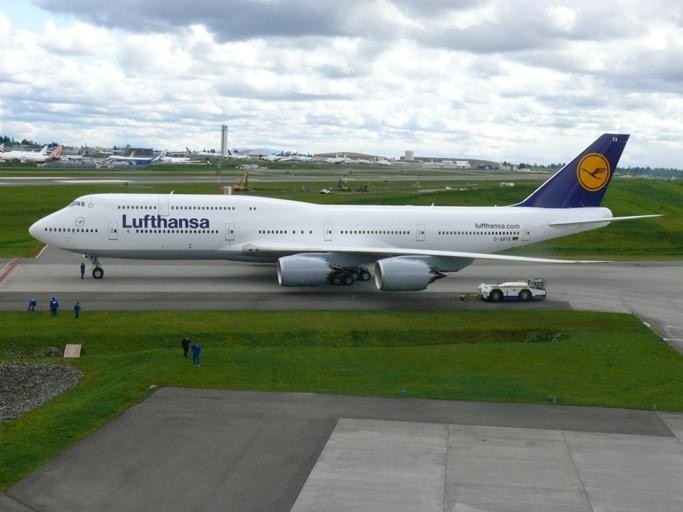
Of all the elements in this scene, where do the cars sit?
[320,188,331,194]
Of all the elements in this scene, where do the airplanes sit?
[27,133,666,292]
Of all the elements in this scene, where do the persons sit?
[73,301,81,317]
[190,340,201,367]
[27,297,38,311]
[338,173,371,192]
[50,296,59,317]
[181,334,190,357]
[80,260,85,279]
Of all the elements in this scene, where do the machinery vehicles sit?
[476,277,547,303]
